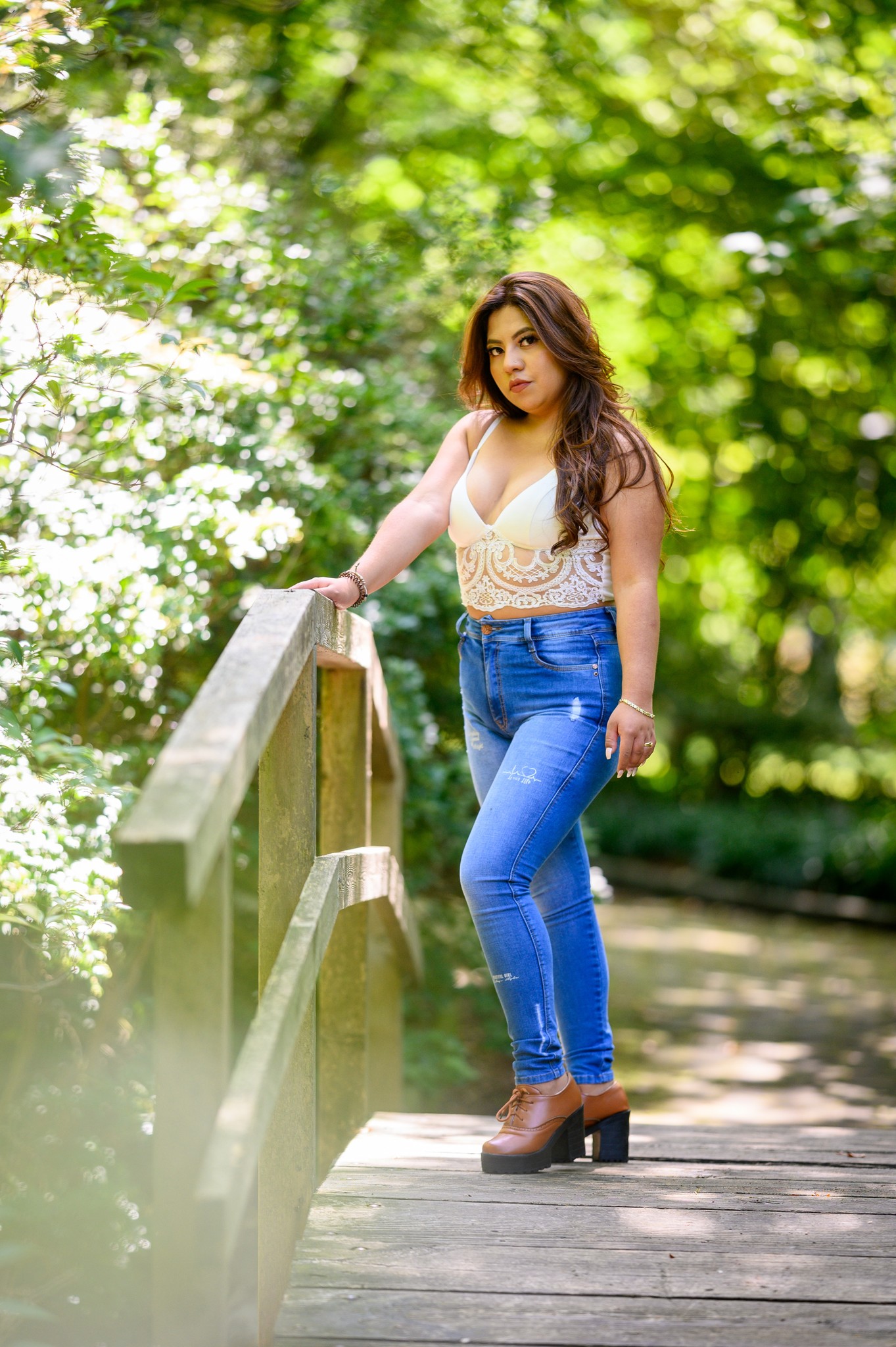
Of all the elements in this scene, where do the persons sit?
[284,272,694,1175]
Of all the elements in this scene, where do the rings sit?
[644,741,653,748]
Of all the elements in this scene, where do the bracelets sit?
[618,699,655,719]
[338,562,368,608]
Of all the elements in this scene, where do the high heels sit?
[481,1071,586,1174]
[582,1082,631,1163]
